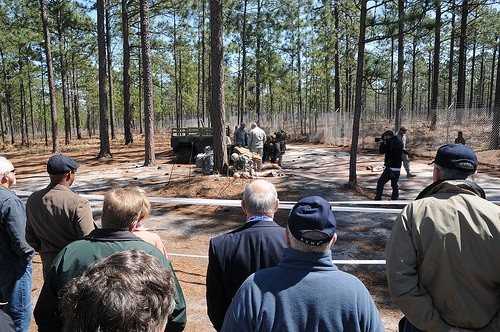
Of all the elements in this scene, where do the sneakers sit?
[407,174,416,178]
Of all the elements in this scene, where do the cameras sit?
[375,138,383,142]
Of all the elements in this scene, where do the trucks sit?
[170,124,231,165]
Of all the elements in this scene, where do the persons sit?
[202,122,286,176]
[0,153,187,332]
[221,195,384,332]
[394,127,416,178]
[371,130,404,206]
[206,179,288,332]
[386,143,500,332]
[455,131,465,145]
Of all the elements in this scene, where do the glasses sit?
[400,129,407,132]
[10,170,14,173]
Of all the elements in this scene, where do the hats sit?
[47,153,80,175]
[251,123,257,128]
[427,143,477,172]
[287,196,336,246]
[400,126,409,131]
[0,157,14,177]
[241,123,246,126]
